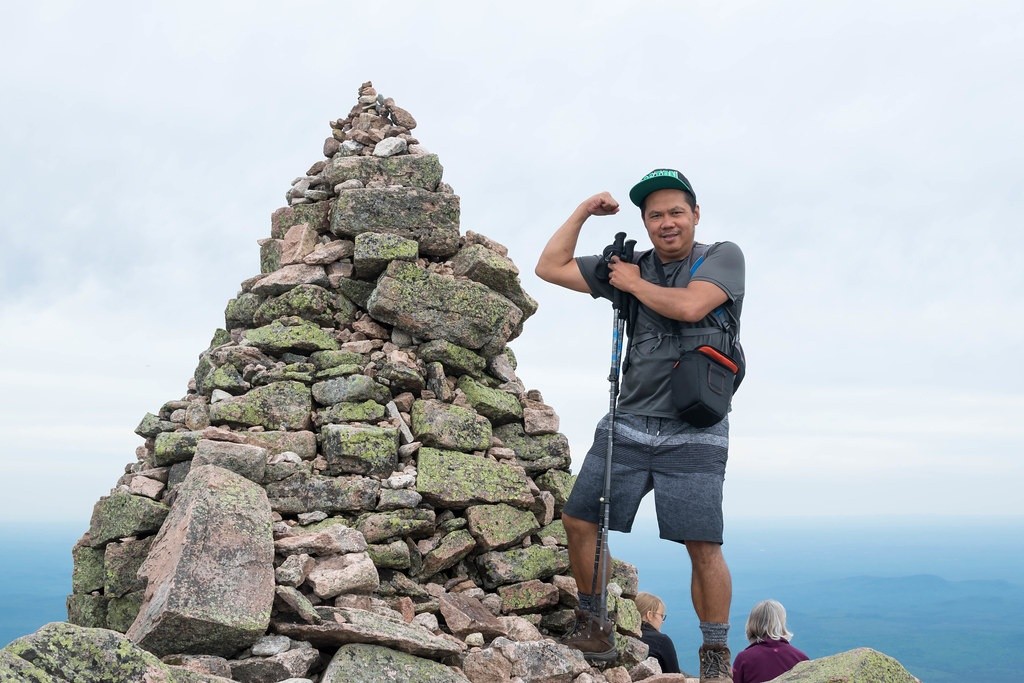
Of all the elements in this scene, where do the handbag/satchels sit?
[671,344,740,429]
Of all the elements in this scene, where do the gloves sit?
[595,232,637,281]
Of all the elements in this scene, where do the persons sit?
[633,592,681,673]
[535,168,746,683]
[732,600,809,683]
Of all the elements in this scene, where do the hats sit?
[629,168,696,209]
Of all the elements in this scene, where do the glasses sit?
[651,611,667,622]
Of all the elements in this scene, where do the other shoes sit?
[559,606,618,661]
[699,643,734,683]
